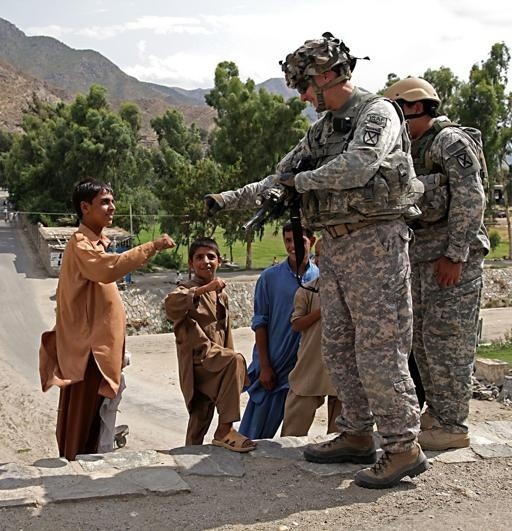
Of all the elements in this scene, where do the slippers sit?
[212,429,256,452]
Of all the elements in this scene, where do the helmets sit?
[382,77,442,110]
[282,33,358,88]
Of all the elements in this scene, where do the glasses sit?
[296,80,310,93]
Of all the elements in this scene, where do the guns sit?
[241,156,314,231]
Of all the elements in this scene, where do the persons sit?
[382,76,492,490]
[40,177,176,461]
[165,237,256,452]
[203,33,430,489]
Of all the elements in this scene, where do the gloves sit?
[205,194,224,216]
[279,172,295,188]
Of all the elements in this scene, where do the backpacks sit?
[457,125,490,205]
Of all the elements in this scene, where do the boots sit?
[304,432,376,465]
[415,411,469,450]
[354,446,430,489]
